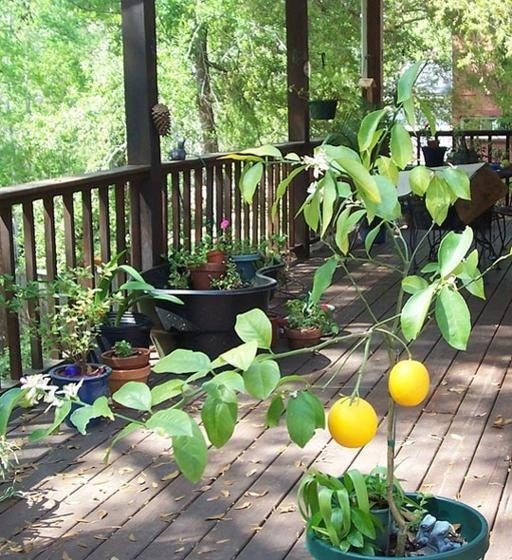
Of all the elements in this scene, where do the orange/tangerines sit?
[388,360,430,407]
[327,397,379,448]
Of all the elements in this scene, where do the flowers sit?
[0,51,512,559]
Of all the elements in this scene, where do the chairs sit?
[340,152,512,279]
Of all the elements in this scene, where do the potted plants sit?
[448,120,481,166]
[289,80,347,122]
[290,459,421,557]
[71,218,339,410]
[420,122,448,167]
[314,91,409,247]
[2,264,112,429]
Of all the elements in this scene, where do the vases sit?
[295,487,491,560]
